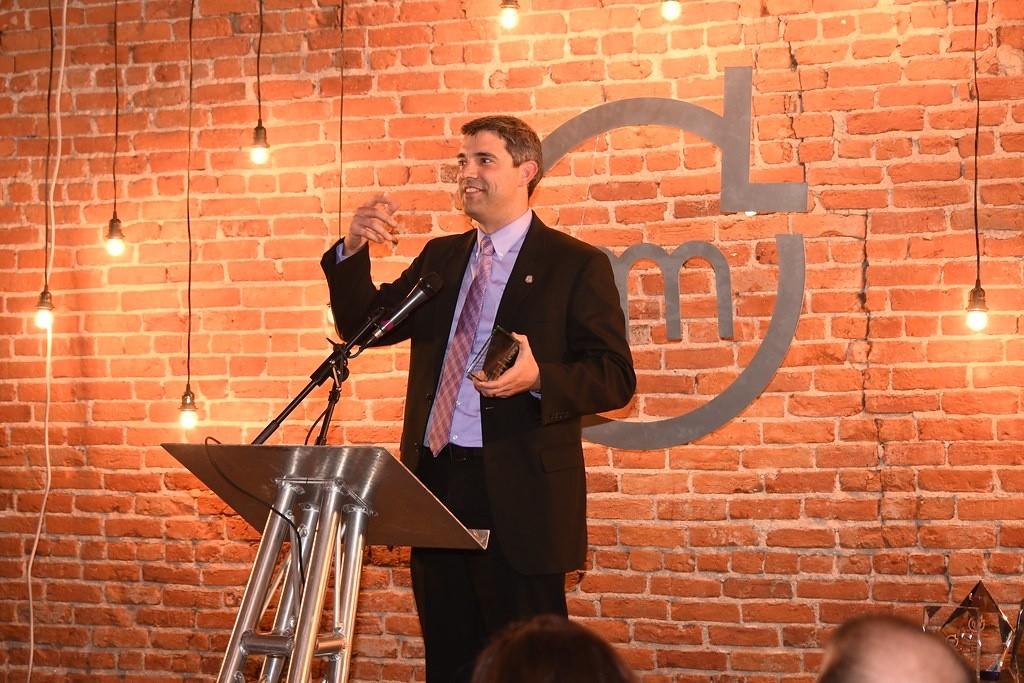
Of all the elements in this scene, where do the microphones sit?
[359,272,443,353]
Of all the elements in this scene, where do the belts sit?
[422,444,483,464]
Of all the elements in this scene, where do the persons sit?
[467,614,639,683]
[322,115,639,683]
[812,614,972,683]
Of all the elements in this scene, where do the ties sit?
[427,237,499,457]
